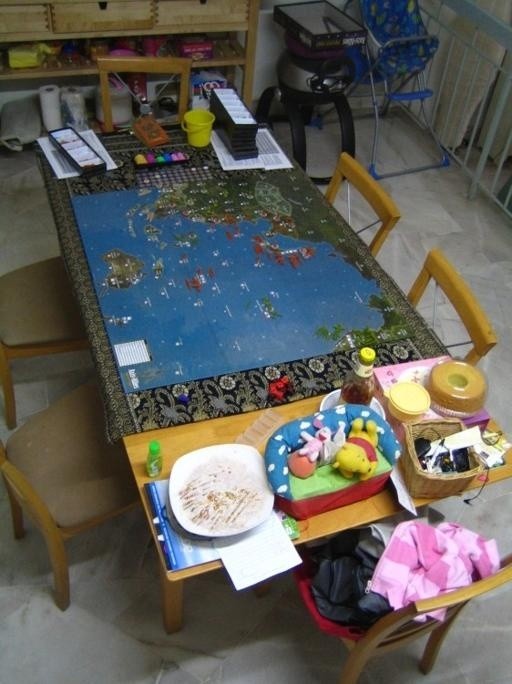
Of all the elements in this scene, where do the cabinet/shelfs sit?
[0,0,260,109]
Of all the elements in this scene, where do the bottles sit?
[145,440,163,480]
[336,346,378,407]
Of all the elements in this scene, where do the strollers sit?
[309,0,451,181]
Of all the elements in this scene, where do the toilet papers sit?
[61,86,83,125]
[39,85,62,131]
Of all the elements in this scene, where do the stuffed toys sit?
[264,404,404,521]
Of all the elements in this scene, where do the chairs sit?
[0,383,142,612]
[96,56,192,132]
[294,531,512,684]
[407,249,498,367]
[0,255,90,430]
[325,153,401,259]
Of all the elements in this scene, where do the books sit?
[143,478,301,574]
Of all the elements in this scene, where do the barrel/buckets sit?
[180,108,215,147]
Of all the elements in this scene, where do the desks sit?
[34,123,512,635]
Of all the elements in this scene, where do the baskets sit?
[401,418,484,498]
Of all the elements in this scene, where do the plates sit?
[165,442,277,540]
[319,388,386,422]
